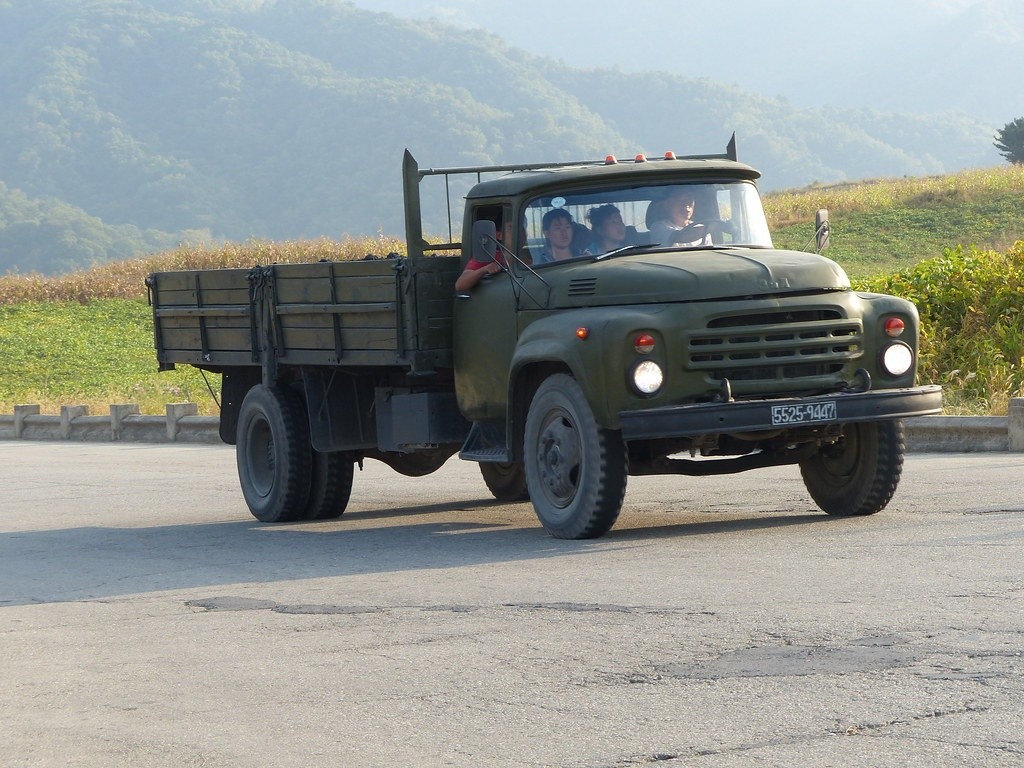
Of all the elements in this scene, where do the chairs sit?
[618,226,640,247]
[644,200,667,230]
[546,222,595,250]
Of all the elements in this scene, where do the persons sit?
[456,210,535,291]
[536,204,626,264]
[649,186,737,248]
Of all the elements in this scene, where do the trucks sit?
[148,131,945,539]
